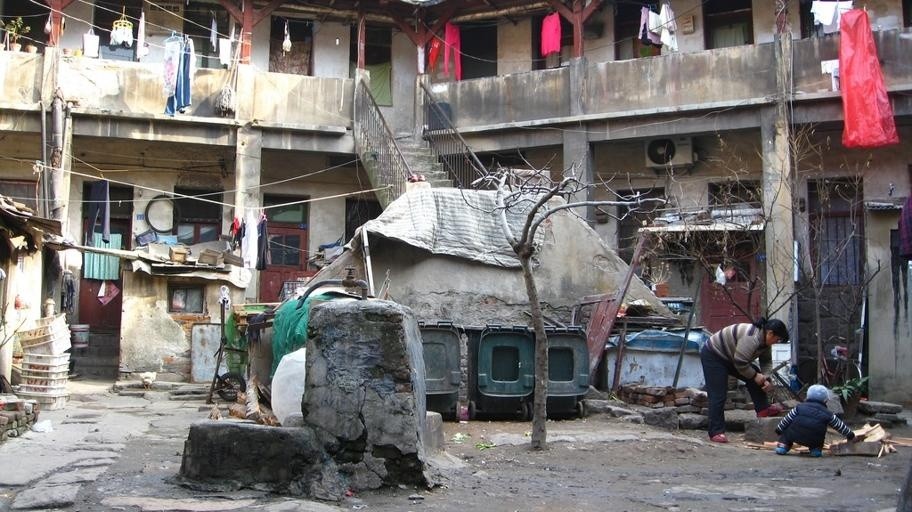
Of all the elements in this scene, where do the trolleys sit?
[204,292,251,407]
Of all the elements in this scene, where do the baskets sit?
[17,351,72,393]
[15,311,73,355]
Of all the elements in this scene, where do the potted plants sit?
[4,16,31,51]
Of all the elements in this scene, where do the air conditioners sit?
[643,134,694,170]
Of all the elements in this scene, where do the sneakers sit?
[775,446,787,455]
[710,434,729,443]
[809,448,822,456]
[755,403,782,417]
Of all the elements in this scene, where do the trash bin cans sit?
[417,320,591,423]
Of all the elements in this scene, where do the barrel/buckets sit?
[69,324,91,349]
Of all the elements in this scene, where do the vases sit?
[64,48,72,56]
[25,45,38,54]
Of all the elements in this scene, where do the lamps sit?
[335,36,340,46]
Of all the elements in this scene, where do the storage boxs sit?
[199,248,224,266]
[169,246,187,262]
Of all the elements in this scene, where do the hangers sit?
[208,9,217,19]
[162,30,190,47]
[87,24,94,35]
[641,0,670,14]
[546,7,560,16]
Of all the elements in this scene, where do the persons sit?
[700,317,790,443]
[774,385,857,457]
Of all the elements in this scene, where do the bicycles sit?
[797,331,865,390]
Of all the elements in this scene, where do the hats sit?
[807,384,830,404]
